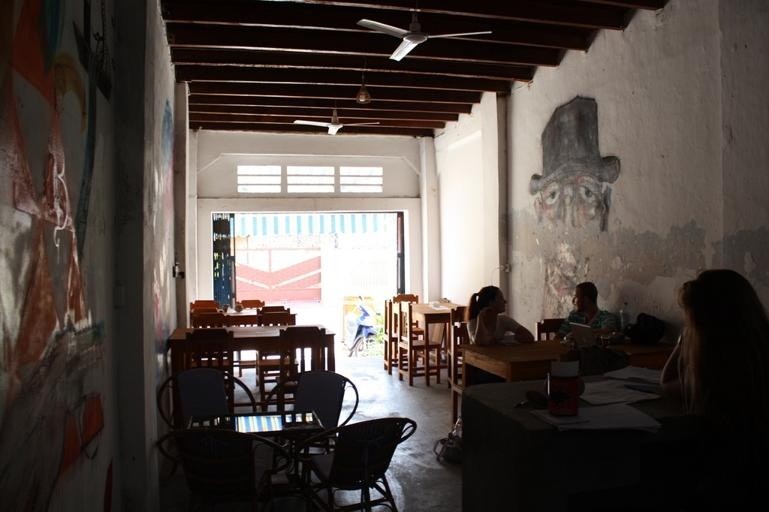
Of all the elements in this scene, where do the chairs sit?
[168,301,335,371]
[264,370,360,426]
[294,416,415,512]
[155,426,292,508]
[379,290,468,387]
[153,367,259,424]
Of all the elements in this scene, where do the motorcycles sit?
[340,296,380,359]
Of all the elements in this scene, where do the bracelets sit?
[678,334,682,345]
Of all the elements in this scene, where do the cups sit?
[504,331,516,344]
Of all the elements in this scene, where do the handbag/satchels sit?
[433,432,463,458]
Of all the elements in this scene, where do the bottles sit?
[620,301,632,328]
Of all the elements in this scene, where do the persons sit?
[659,269,769,512]
[554,282,621,376]
[467,287,534,383]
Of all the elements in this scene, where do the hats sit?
[528,96,620,195]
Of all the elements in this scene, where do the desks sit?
[453,338,667,423]
[460,365,691,511]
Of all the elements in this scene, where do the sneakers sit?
[452,418,463,439]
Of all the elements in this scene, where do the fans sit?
[293,109,382,138]
[356,11,495,65]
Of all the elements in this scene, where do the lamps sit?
[355,71,374,106]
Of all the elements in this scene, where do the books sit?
[568,322,592,337]
